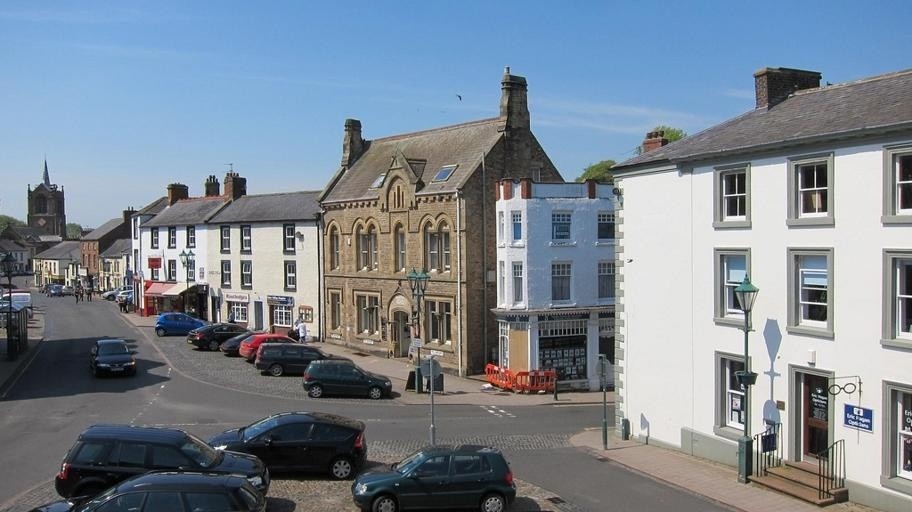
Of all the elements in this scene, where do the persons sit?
[23,277,27,288]
[79,286,84,302]
[294,315,302,327]
[118,296,123,313]
[86,285,92,302]
[229,310,235,323]
[123,296,130,313]
[294,319,308,344]
[73,283,80,304]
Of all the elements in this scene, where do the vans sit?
[255,343,354,377]
[0,288,34,328]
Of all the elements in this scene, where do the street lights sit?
[2,253,17,362]
[407,268,431,392]
[178,248,196,314]
[735,275,759,483]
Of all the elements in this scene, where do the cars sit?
[350,444,517,511]
[302,359,392,399]
[88,339,136,377]
[102,284,133,303]
[155,312,205,337]
[41,283,75,297]
[209,411,368,479]
[220,330,269,355]
[187,323,251,351]
[239,333,300,359]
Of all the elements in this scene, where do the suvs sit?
[29,471,267,512]
[55,424,270,498]
